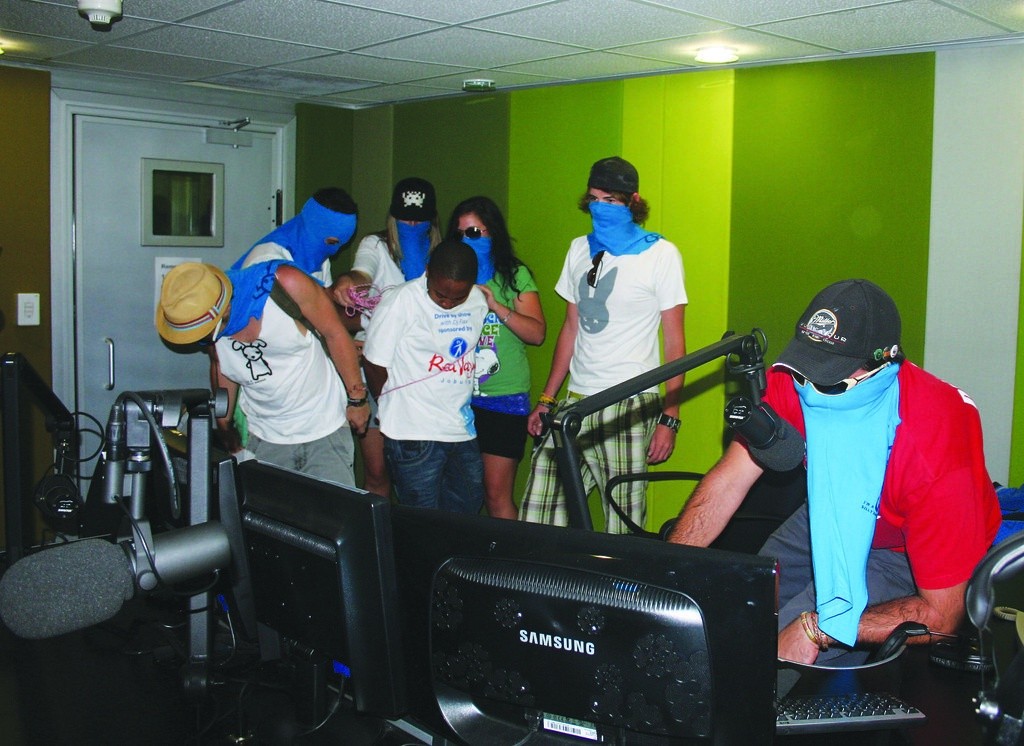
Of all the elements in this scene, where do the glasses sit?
[455,226,488,242]
[792,361,892,397]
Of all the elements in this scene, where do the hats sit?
[154,262,233,344]
[388,177,437,222]
[588,156,638,196]
[773,280,902,386]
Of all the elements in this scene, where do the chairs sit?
[964,528,1024,746]
[605,429,806,555]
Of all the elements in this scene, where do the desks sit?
[0,612,1024,746]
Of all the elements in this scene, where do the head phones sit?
[861,619,998,674]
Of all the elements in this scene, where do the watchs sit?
[657,413,681,432]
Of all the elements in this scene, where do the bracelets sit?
[801,609,839,649]
[502,307,511,324]
[539,392,557,408]
[348,392,371,406]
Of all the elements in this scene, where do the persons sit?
[665,277,1004,666]
[209,185,368,430]
[156,261,370,487]
[356,241,488,520]
[517,156,690,536]
[446,197,547,518]
[353,176,444,503]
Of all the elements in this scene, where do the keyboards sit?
[775,691,926,737]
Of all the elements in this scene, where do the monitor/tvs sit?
[150,426,781,746]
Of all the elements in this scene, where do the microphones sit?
[725,396,805,471]
[75,401,125,543]
[0,519,231,641]
